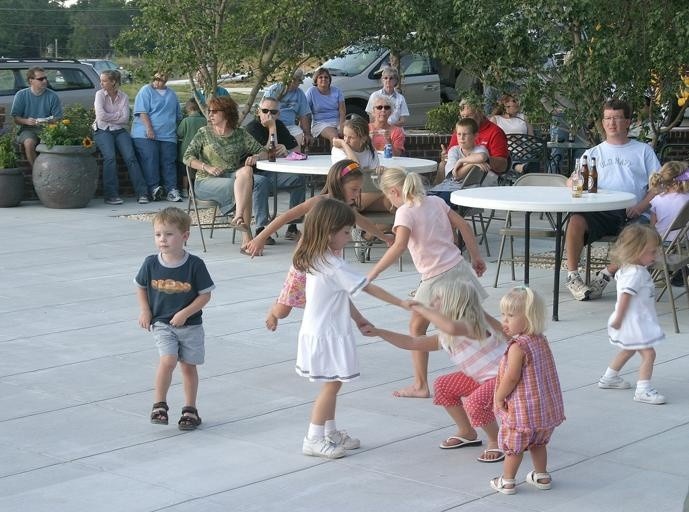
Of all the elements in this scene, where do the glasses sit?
[260,108,281,114]
[33,76,47,81]
[208,109,224,114]
[376,105,391,110]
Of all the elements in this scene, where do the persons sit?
[598,223,669,404]
[183,64,409,255]
[91,68,232,203]
[565,99,664,300]
[246,159,565,494]
[11,66,63,166]
[134,206,216,429]
[428,94,544,251]
[648,162,689,286]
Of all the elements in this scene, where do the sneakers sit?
[632,388,666,405]
[564,273,592,302]
[254,233,276,244]
[588,271,610,299]
[105,186,188,204]
[324,428,360,451]
[284,230,301,240]
[302,437,347,459]
[351,228,371,263]
[598,375,631,390]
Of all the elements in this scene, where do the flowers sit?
[39,105,96,150]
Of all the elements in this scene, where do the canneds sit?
[384,144,393,157]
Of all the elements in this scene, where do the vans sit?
[263,30,531,132]
[2,57,103,134]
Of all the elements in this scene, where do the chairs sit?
[404,51,428,75]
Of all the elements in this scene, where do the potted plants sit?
[0,133,24,208]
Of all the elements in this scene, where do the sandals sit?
[490,474,516,495]
[150,402,168,424]
[526,470,552,490]
[178,406,201,429]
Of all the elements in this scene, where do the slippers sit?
[239,244,262,256]
[477,448,504,462]
[230,215,249,232]
[440,436,482,450]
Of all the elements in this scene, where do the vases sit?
[32,144,98,208]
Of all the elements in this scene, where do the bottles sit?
[268,133,276,162]
[300,133,308,160]
[571,153,598,198]
[551,124,558,143]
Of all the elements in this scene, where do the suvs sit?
[56,59,133,85]
